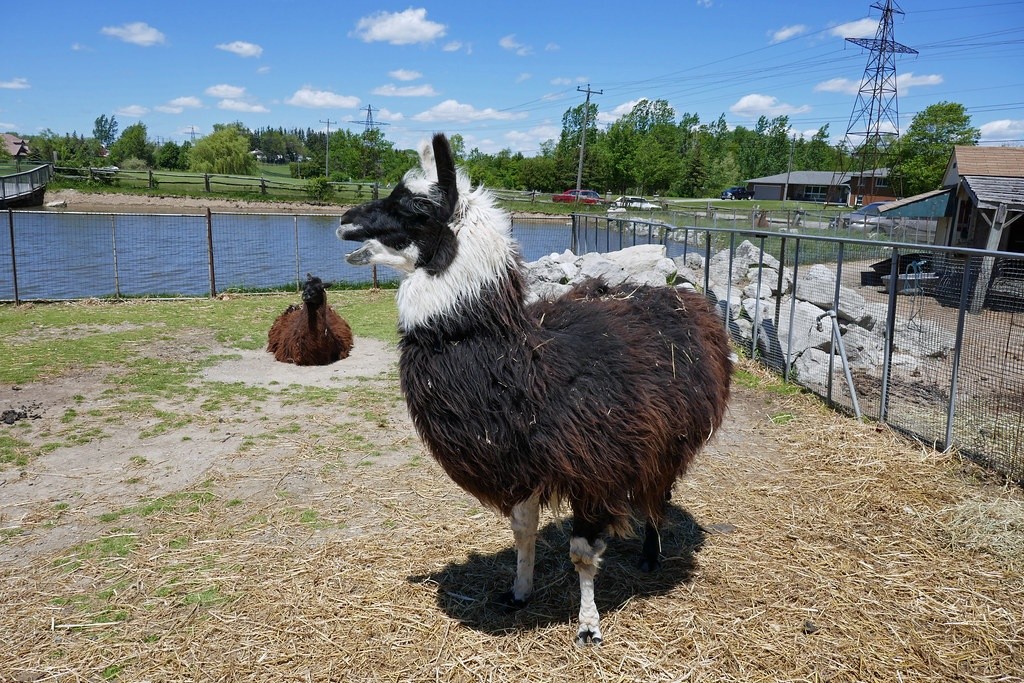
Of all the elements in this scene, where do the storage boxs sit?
[880,272,939,292]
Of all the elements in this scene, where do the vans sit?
[828,202,938,244]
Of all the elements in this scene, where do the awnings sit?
[860,183,959,218]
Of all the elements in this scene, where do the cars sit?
[552,190,608,204]
[611,196,662,210]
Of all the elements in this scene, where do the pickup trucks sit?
[720,186,755,200]
